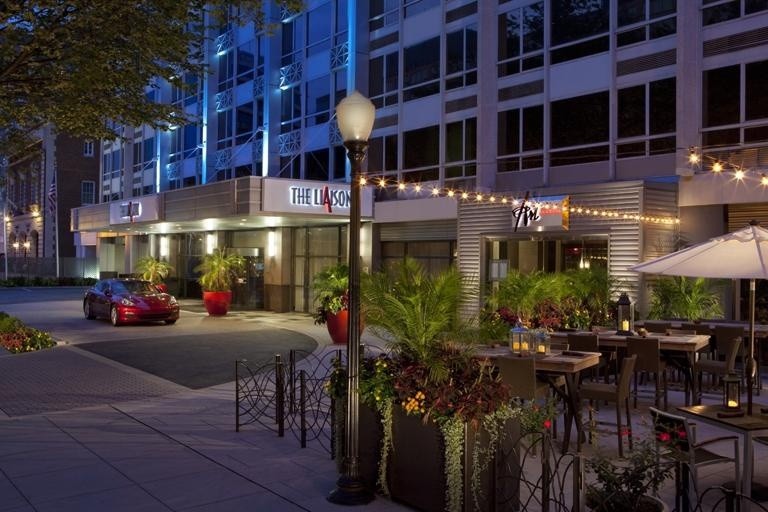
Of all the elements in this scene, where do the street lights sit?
[326,90,376,506]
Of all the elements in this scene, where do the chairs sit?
[571,353,639,458]
[480,323,768,410]
[651,406,741,510]
[492,356,552,458]
[753,434,768,448]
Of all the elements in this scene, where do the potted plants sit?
[194,248,246,317]
[314,267,383,344]
[136,257,174,294]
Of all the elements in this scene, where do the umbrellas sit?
[627,218,768,415]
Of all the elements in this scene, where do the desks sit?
[677,403,767,497]
[479,347,603,444]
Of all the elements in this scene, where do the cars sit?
[83,278,181,326]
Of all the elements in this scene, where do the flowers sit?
[517,397,692,512]
[325,352,510,423]
